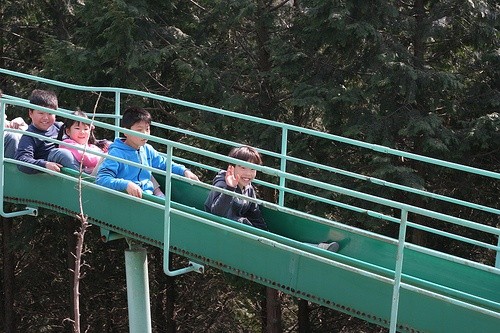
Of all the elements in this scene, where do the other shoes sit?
[318,242,338,252]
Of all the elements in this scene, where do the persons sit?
[56,110,166,200]
[95,105,203,199]
[204,145,340,253]
[4,114,29,159]
[14,89,68,174]
